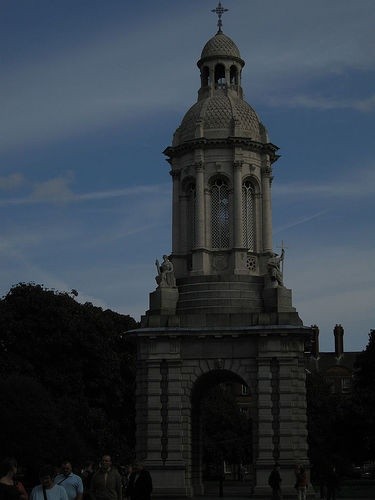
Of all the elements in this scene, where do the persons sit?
[268,461,342,500]
[155,254,174,288]
[266,249,285,288]
[0,452,154,500]
